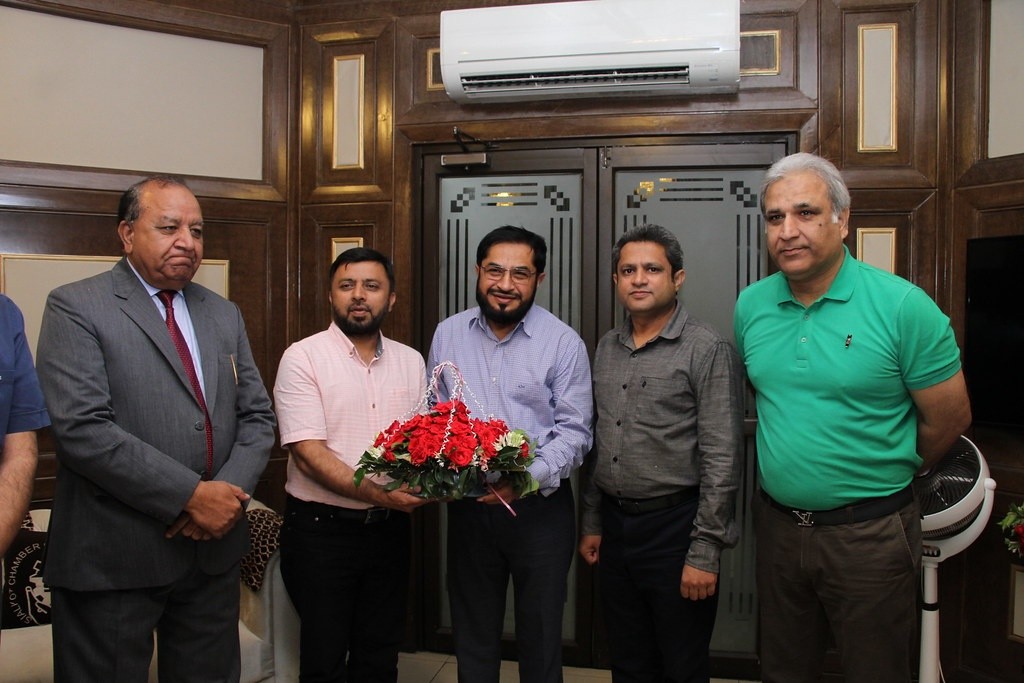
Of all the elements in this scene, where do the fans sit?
[906,435,997,683]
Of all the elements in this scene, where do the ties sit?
[155,289,213,482]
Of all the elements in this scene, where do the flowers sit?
[351,399,545,504]
[997,500,1024,564]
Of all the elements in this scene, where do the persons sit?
[0,295,51,558]
[427,226,593,683]
[578,224,743,683]
[273,247,437,683]
[36,174,277,683]
[733,153,972,683]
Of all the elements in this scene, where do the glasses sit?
[479,266,538,284]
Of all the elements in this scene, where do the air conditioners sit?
[439,0,742,107]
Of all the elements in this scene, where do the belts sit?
[288,496,401,526]
[759,485,913,527]
[602,486,700,517]
